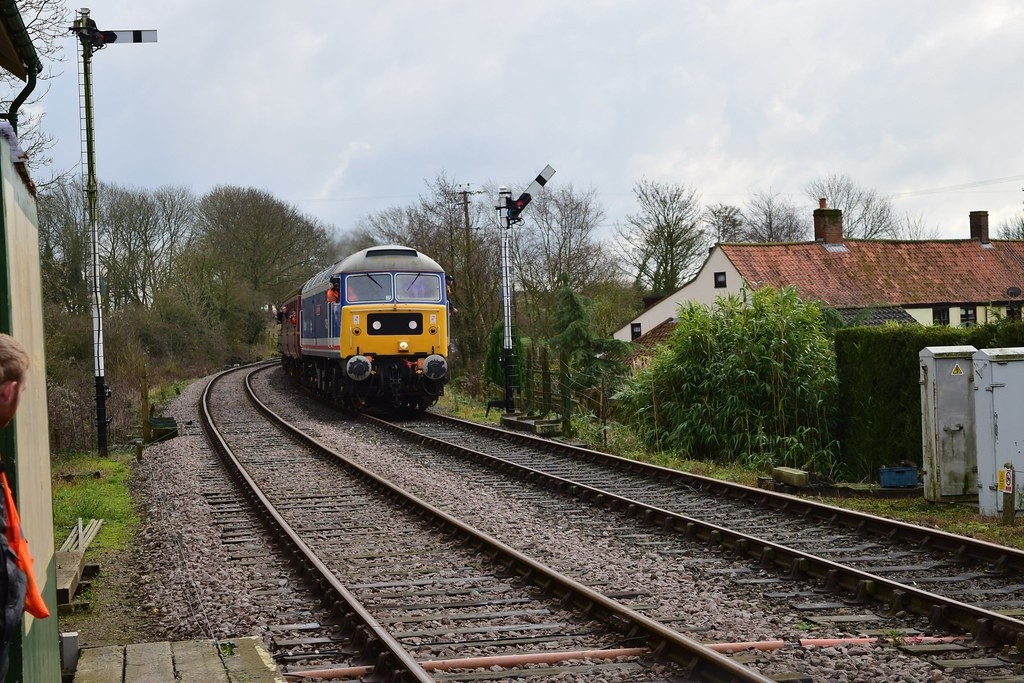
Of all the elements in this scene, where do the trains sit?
[271,241,448,415]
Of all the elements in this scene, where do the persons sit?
[0,333,49,683]
[444,275,457,313]
[326,281,342,304]
[272,307,297,324]
